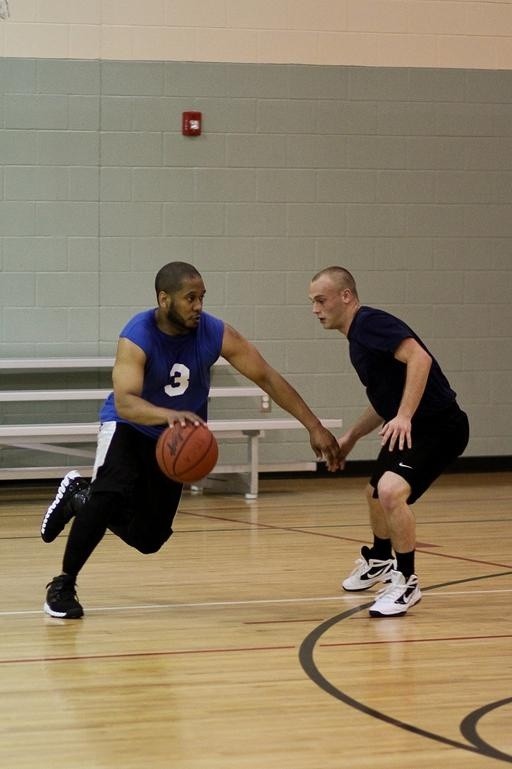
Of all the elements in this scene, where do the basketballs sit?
[156,419,219,482]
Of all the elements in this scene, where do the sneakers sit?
[43,573,84,619]
[40,470,94,543]
[368,569,423,618]
[341,545,398,592]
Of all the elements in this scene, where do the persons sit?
[40,262,343,618]
[308,266,469,617]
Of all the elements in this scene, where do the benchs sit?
[1,353,341,502]
